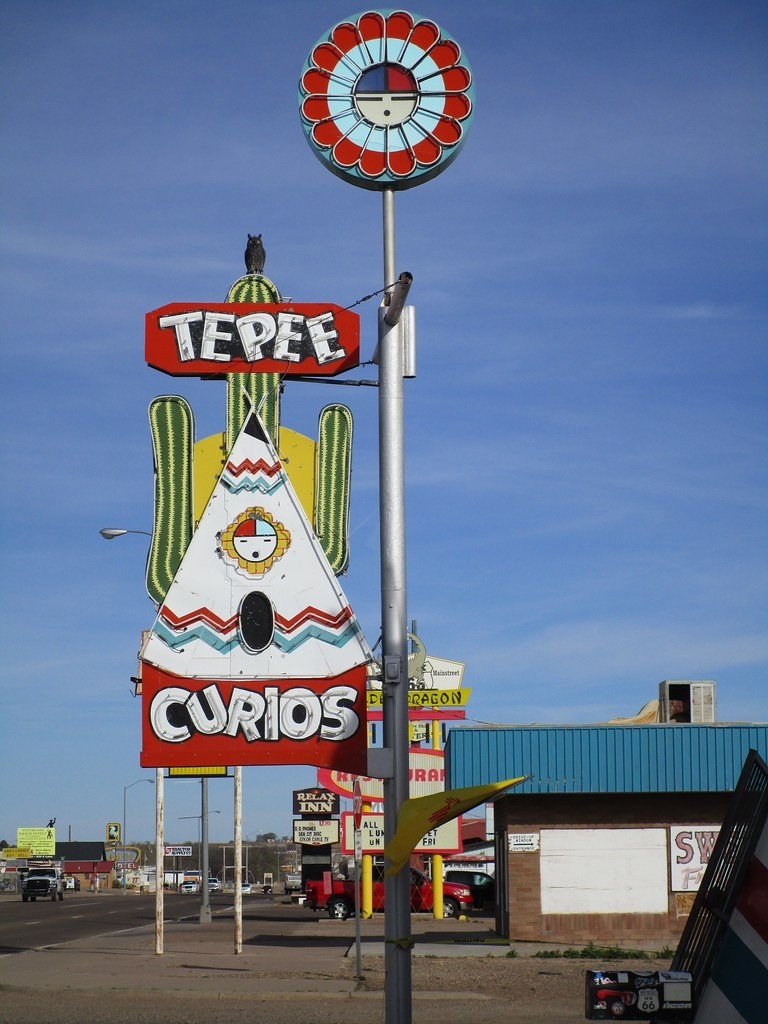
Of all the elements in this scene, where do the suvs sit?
[20,868,64,901]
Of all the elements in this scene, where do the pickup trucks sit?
[444,870,496,909]
[303,864,474,920]
[284,876,302,895]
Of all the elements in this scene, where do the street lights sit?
[178,810,221,881]
[100,527,243,954]
[123,779,155,895]
[246,831,259,884]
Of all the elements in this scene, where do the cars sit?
[242,883,252,895]
[64,877,80,891]
[181,880,200,892]
[209,878,222,891]
[261,886,273,895]
